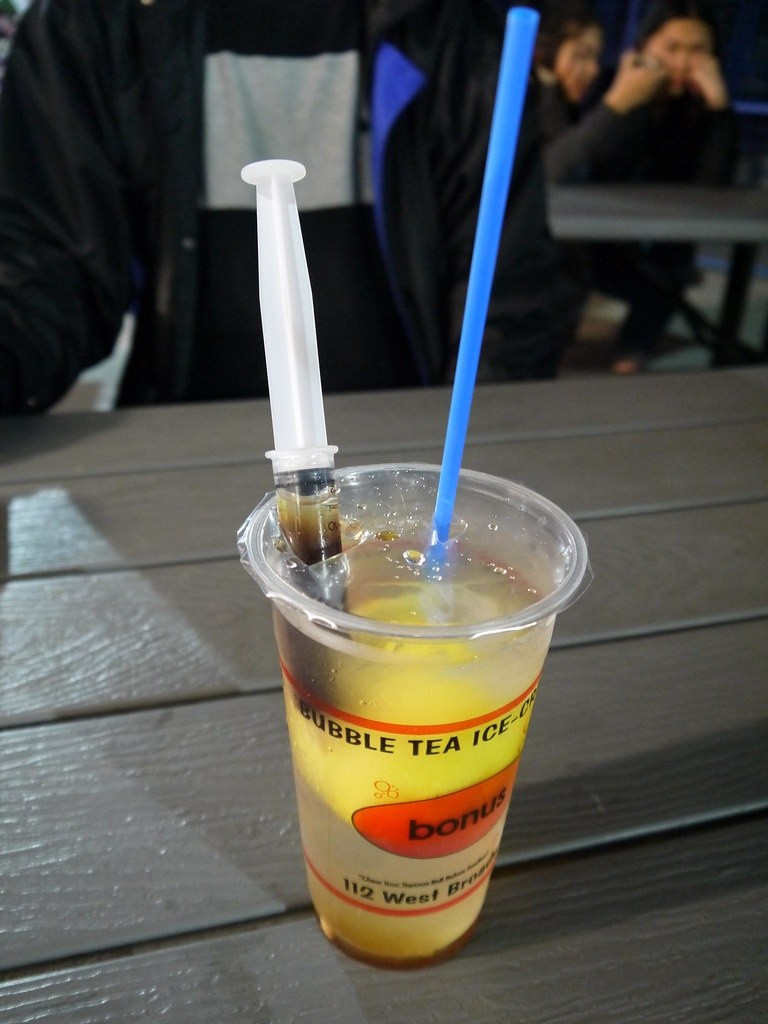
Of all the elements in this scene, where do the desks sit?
[0,367,768,1024]
[550,173,768,367]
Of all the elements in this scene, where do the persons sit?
[0,0,731,415]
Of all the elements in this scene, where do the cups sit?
[237,463,595,970]
